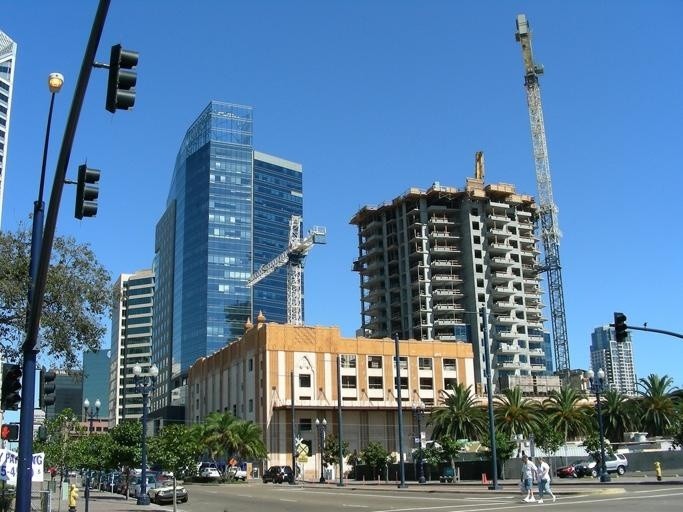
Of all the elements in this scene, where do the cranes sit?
[245,215,328,327]
[509,8,574,382]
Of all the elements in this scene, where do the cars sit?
[66,462,189,502]
[189,460,248,483]
[553,459,585,480]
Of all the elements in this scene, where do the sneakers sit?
[521,496,556,504]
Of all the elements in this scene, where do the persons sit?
[534,455,555,503]
[46,464,60,483]
[520,455,538,502]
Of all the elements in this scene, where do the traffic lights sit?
[37,425,46,441]
[39,365,57,412]
[0,424,18,441]
[1,364,20,411]
[612,311,626,343]
[73,161,100,218]
[105,42,141,111]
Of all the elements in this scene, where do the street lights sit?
[131,361,161,506]
[411,400,427,484]
[14,68,63,512]
[588,365,616,483]
[80,397,100,489]
[314,417,328,484]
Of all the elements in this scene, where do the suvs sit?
[574,451,630,478]
[261,464,294,485]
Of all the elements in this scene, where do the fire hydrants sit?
[653,462,662,482]
[67,483,77,511]
